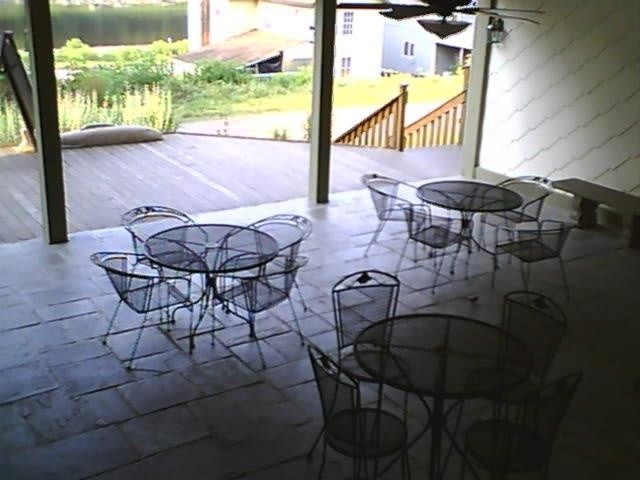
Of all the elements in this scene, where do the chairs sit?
[479,289,569,426]
[492,219,573,299]
[397,203,474,294]
[92,251,194,372]
[477,177,554,265]
[215,213,312,317]
[308,345,412,479]
[305,269,413,463]
[360,174,431,265]
[210,255,309,369]
[123,205,209,320]
[459,367,583,479]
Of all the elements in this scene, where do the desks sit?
[142,223,279,351]
[354,315,535,478]
[417,180,524,275]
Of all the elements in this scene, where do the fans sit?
[339,0,545,37]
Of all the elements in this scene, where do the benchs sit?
[552,170,640,245]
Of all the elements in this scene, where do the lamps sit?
[417,15,471,38]
[487,17,505,42]
